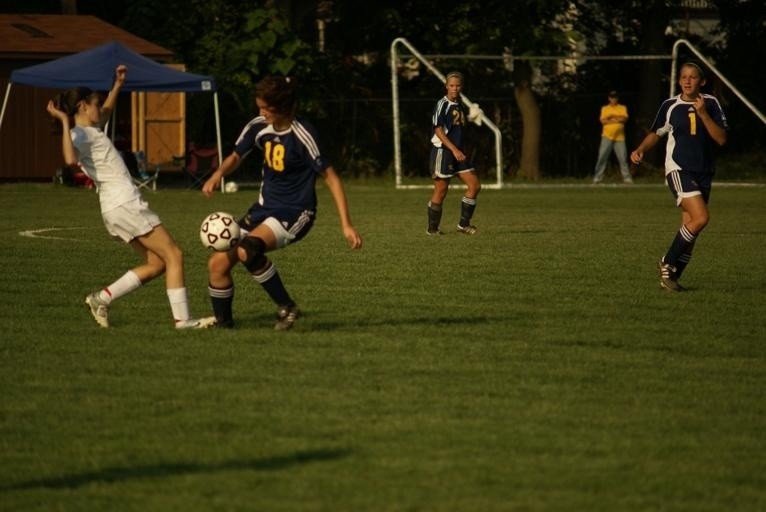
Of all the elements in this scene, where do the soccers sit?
[199,210,240,253]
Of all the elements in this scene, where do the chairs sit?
[130,152,160,192]
[173,142,218,191]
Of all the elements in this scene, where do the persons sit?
[43,64,217,329]
[592,91,635,185]
[425,72,483,236]
[629,62,729,293]
[200,77,362,332]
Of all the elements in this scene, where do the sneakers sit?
[426,224,477,235]
[175,316,216,328]
[274,300,300,331]
[658,255,687,291]
[210,320,235,329]
[86,292,109,329]
